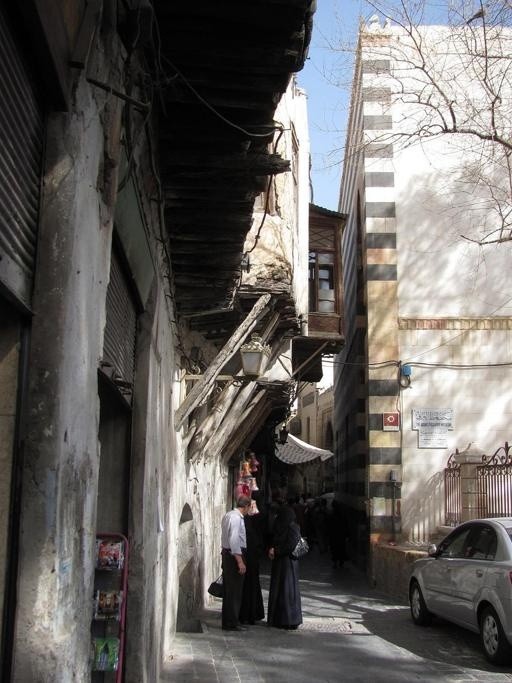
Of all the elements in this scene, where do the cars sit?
[408,516,512,666]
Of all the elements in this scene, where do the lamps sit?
[185,333,272,383]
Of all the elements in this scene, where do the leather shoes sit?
[222,619,298,631]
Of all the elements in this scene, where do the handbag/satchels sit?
[208,575,224,597]
[293,537,310,558]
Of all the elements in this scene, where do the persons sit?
[217,494,253,632]
[236,513,265,624]
[265,504,304,629]
[268,485,352,568]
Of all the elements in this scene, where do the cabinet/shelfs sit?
[93,531,129,683]
